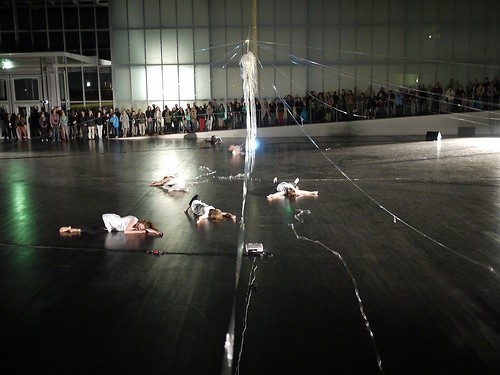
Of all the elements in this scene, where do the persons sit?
[0,76,500,156]
[59,213,163,238]
[184,195,236,225]
[267,177,318,200]
[148,176,189,193]
